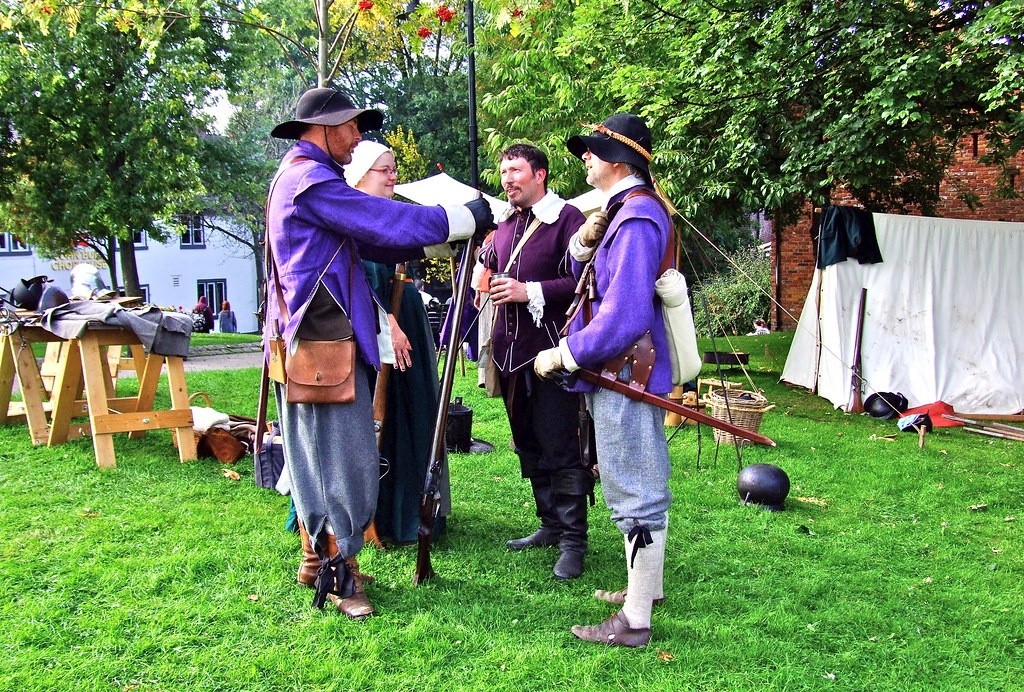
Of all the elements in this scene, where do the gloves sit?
[449,197,498,249]
[533,347,563,383]
[578,212,609,248]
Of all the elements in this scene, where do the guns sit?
[851,287,867,415]
[364,260,409,548]
[413,190,484,586]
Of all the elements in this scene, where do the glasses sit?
[370,168,398,175]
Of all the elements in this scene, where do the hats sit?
[567,114,652,173]
[343,139,390,189]
[270,88,384,140]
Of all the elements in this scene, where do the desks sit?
[0,308,198,470]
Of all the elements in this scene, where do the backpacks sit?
[193,306,210,332]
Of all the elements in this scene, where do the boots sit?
[552,468,595,580]
[326,535,375,618]
[296,519,374,588]
[507,472,560,549]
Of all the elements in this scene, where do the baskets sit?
[170,392,210,449]
[704,389,776,447]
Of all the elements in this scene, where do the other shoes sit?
[594,587,664,608]
[571,608,651,647]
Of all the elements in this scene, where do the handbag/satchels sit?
[285,338,356,403]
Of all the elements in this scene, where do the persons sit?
[289,140,456,546]
[468,145,597,579]
[260,87,494,616]
[218,302,237,333]
[541,111,673,644]
[193,296,214,333]
